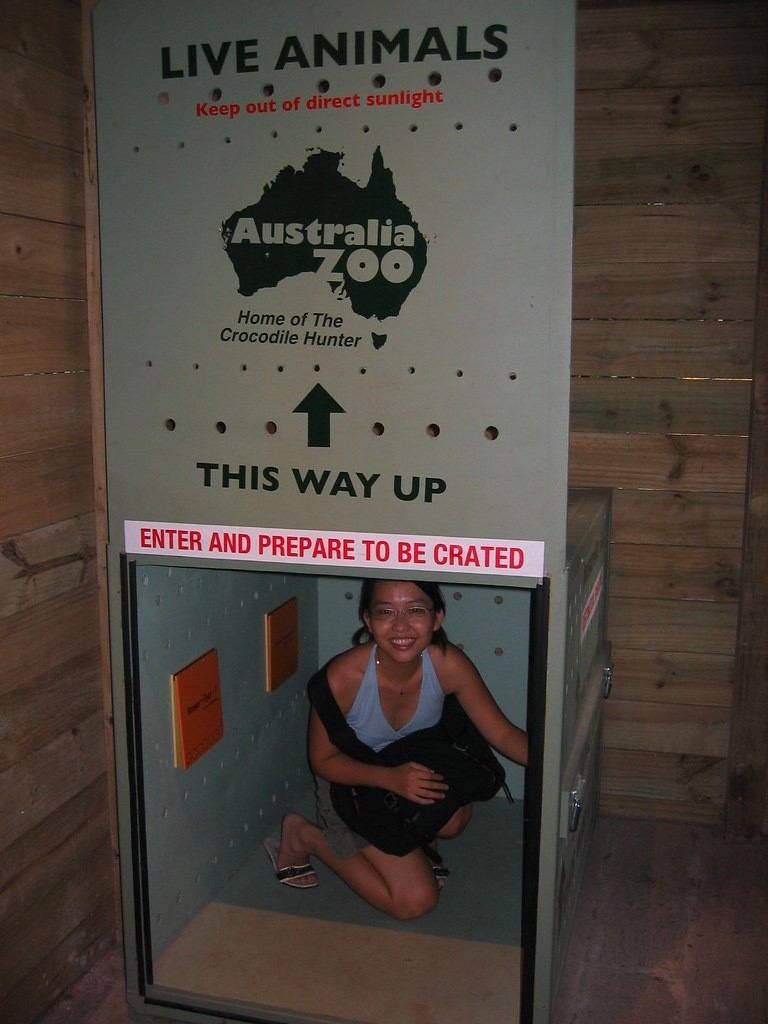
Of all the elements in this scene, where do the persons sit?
[263,578,528,918]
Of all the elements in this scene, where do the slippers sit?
[264,837,317,889]
[433,858,449,893]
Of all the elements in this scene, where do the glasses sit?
[368,607,435,622]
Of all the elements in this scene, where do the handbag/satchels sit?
[335,719,507,857]
